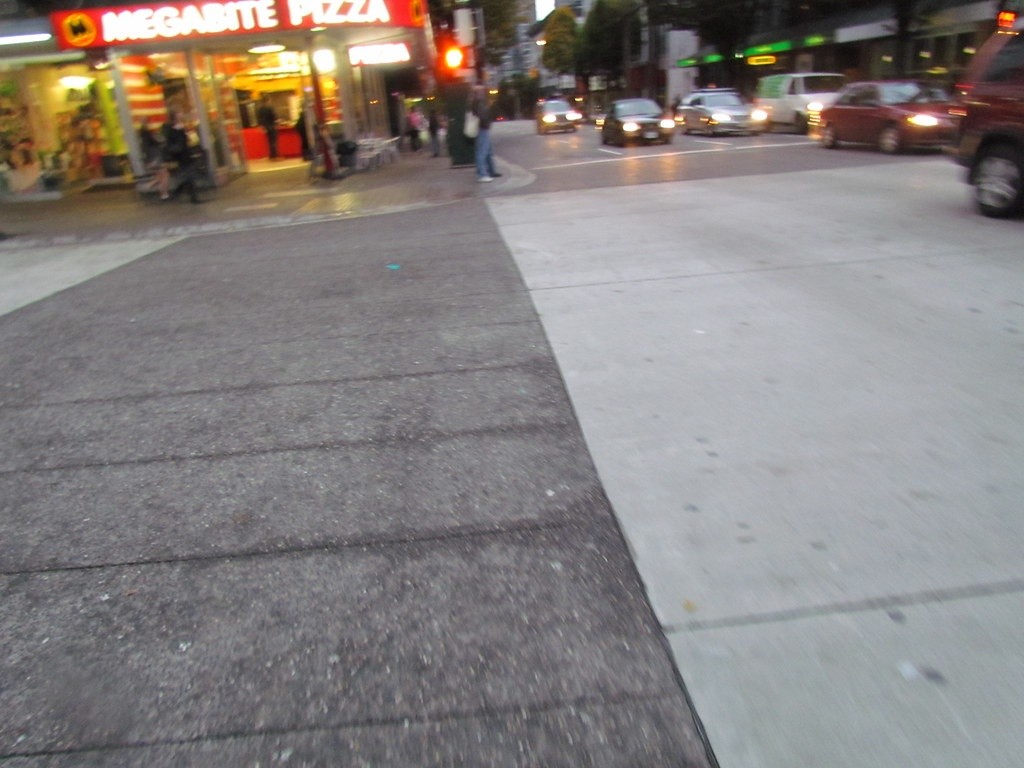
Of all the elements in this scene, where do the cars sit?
[942,14,1024,220]
[674,89,768,137]
[536,100,580,134]
[809,81,964,152]
[600,98,675,147]
[753,74,851,133]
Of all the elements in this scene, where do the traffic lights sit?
[445,48,463,70]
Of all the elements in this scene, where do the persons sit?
[136,118,169,201]
[298,104,313,160]
[407,106,422,153]
[163,110,204,204]
[471,85,502,184]
[259,96,277,157]
[429,110,440,156]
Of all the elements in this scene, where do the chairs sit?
[353,134,400,170]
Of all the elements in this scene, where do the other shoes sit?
[489,171,503,178]
[476,175,492,182]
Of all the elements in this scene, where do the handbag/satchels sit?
[464,111,480,138]
